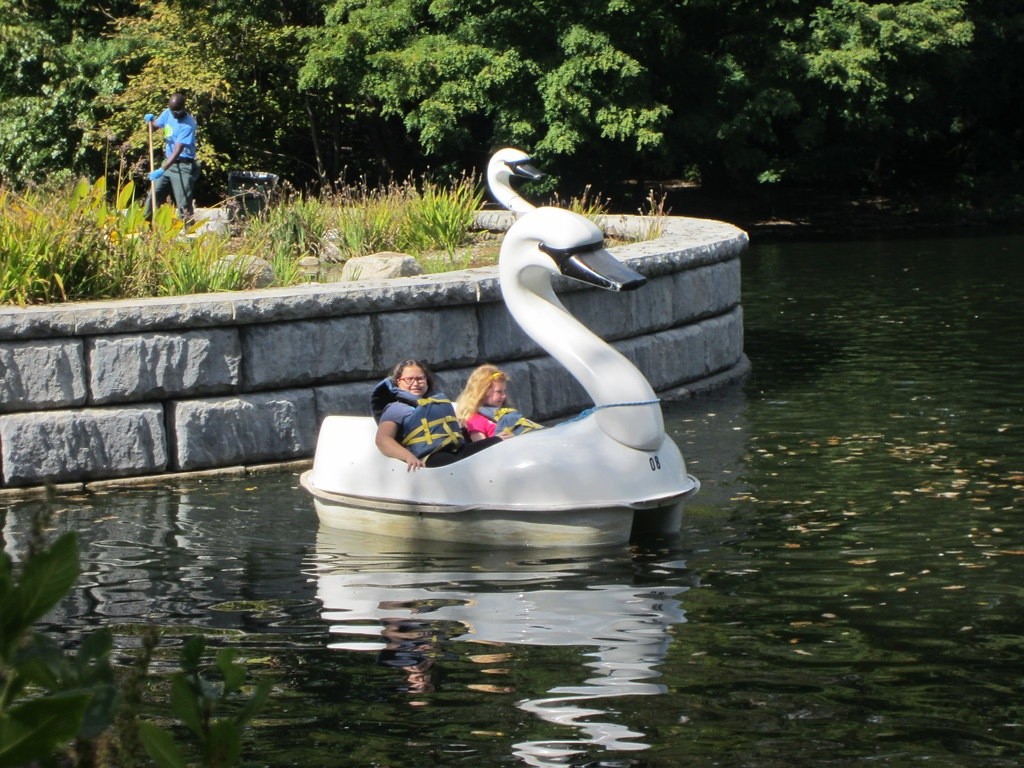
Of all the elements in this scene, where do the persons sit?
[143,94,197,234]
[375,359,544,472]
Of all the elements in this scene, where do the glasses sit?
[398,376,426,385]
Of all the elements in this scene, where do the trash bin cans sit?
[227,170,280,238]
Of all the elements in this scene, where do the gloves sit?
[144,113,154,122]
[148,167,164,181]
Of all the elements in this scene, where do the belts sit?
[174,159,194,163]
